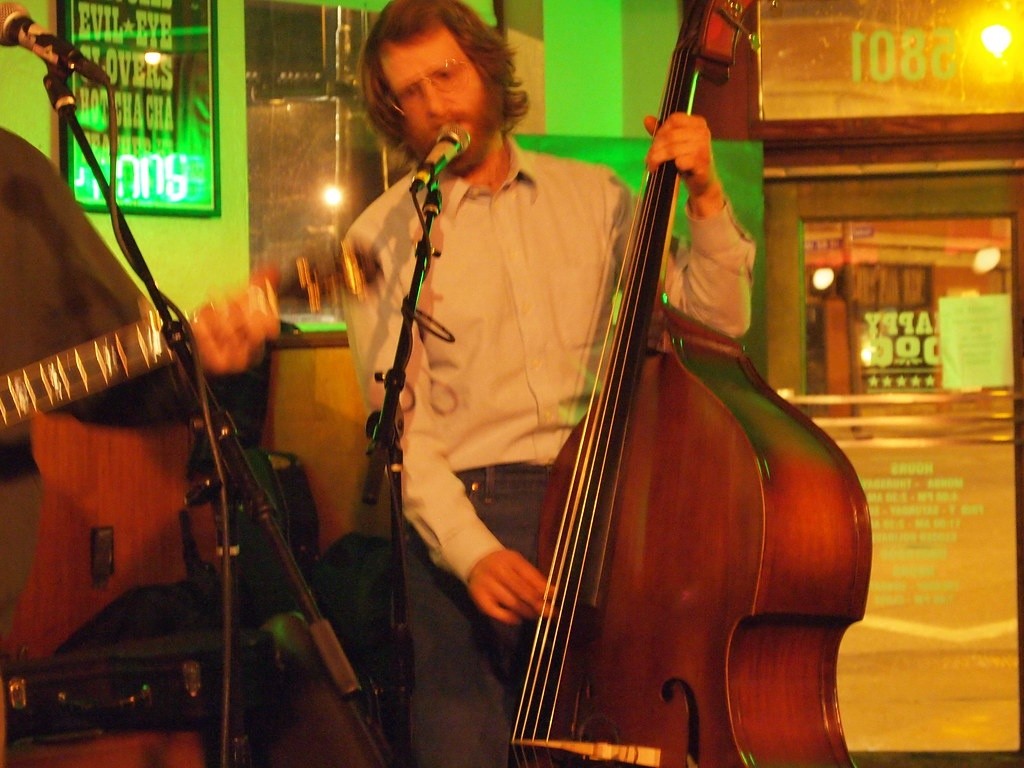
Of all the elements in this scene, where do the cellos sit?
[507,1,873,768]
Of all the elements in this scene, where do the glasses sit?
[388,58,475,116]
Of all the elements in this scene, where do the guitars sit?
[1,229,384,424]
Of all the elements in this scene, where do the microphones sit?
[0,3,111,86]
[409,124,470,193]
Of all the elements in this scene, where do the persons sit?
[338,0,760,768]
[0,128,281,484]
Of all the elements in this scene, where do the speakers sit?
[260,327,393,561]
